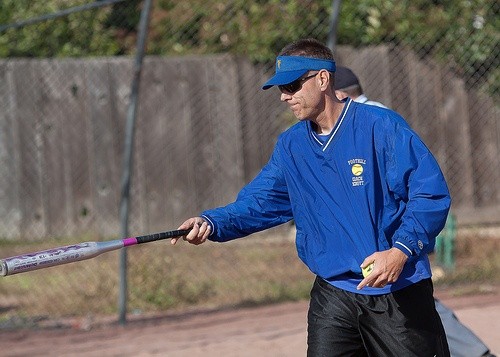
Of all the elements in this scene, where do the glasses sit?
[277,73,318,93]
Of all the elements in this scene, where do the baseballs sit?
[360,262,379,279]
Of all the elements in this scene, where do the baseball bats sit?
[0,228,196,278]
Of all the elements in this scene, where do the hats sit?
[332,65,359,90]
[262,56,336,90]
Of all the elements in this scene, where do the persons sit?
[170,37,451,356]
[332,65,496,356]
